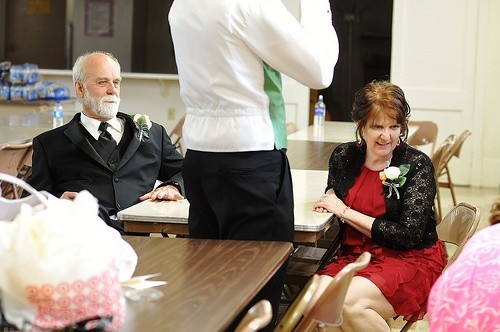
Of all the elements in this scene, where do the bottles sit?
[53,101,63,129]
[0,62,68,102]
[313,95,326,128]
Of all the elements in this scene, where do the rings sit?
[321,196,326,201]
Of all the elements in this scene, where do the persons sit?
[168,0,340,332]
[19,51,186,237]
[303,81,449,332]
[428,199,500,332]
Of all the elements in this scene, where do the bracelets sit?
[340,206,351,223]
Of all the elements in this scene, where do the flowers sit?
[134,113,153,142]
[377,165,411,194]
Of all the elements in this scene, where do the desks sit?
[1,108,80,145]
[0,97,77,112]
[285,120,419,144]
[113,235,295,332]
[284,138,339,171]
[116,168,337,244]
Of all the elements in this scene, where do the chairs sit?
[0,139,53,218]
[170,116,185,157]
[407,119,472,223]
[234,202,482,332]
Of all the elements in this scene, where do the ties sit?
[97,122,117,157]
